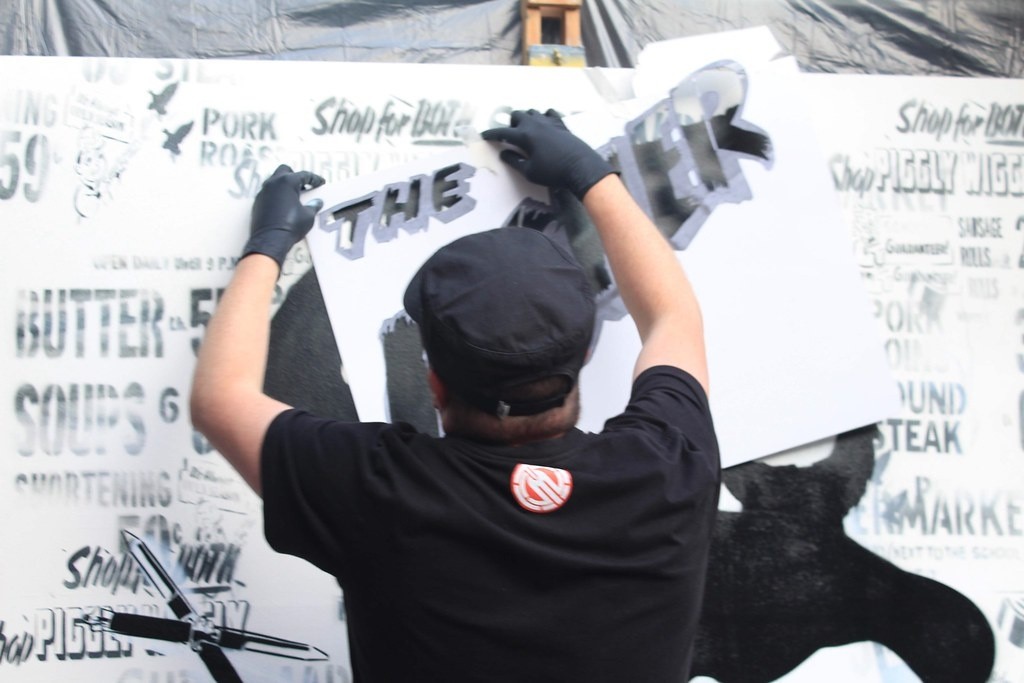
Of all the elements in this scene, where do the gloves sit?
[235,164,325,278]
[480,109,621,198]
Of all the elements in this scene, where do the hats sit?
[404,224,595,416]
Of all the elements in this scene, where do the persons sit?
[188,109,725,682]
